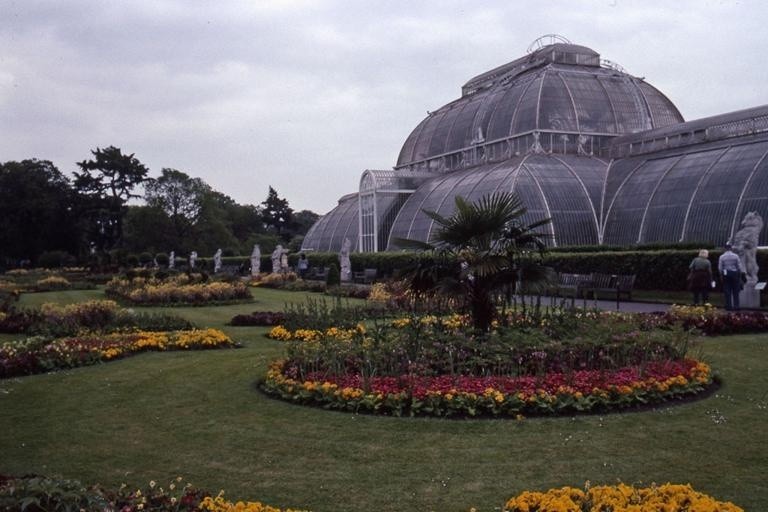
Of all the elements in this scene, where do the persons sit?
[690,249,714,304]
[717,243,747,310]
[296,253,308,279]
